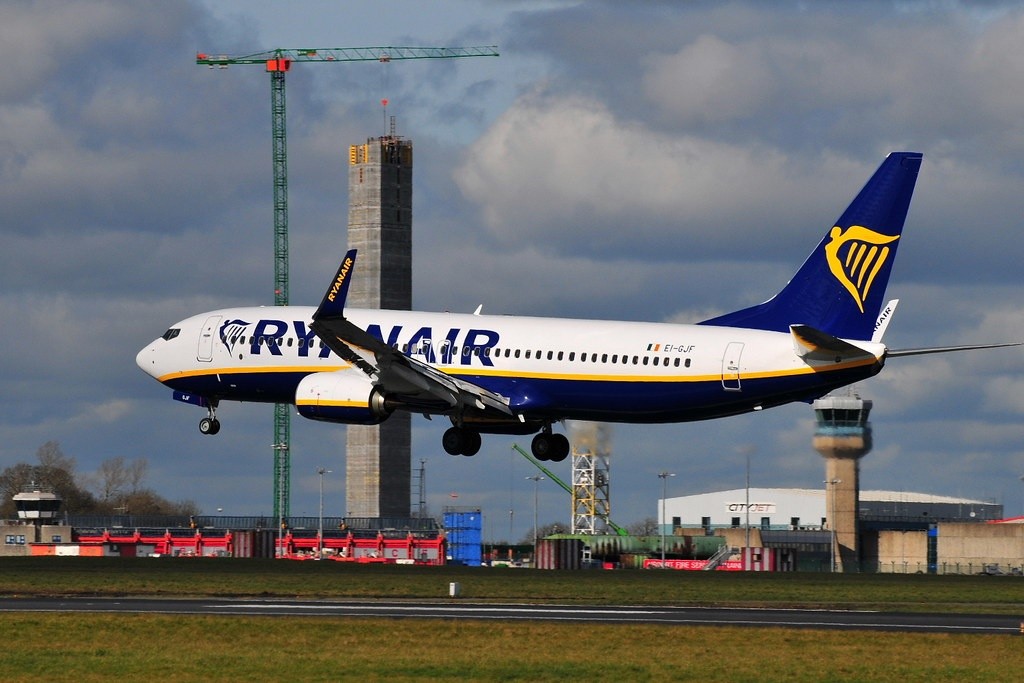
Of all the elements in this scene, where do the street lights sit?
[525,475,544,568]
[316,467,333,560]
[658,472,677,570]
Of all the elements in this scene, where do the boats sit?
[12,469,64,519]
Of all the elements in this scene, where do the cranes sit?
[193,45,500,533]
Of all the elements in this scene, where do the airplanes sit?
[134,150,924,460]
